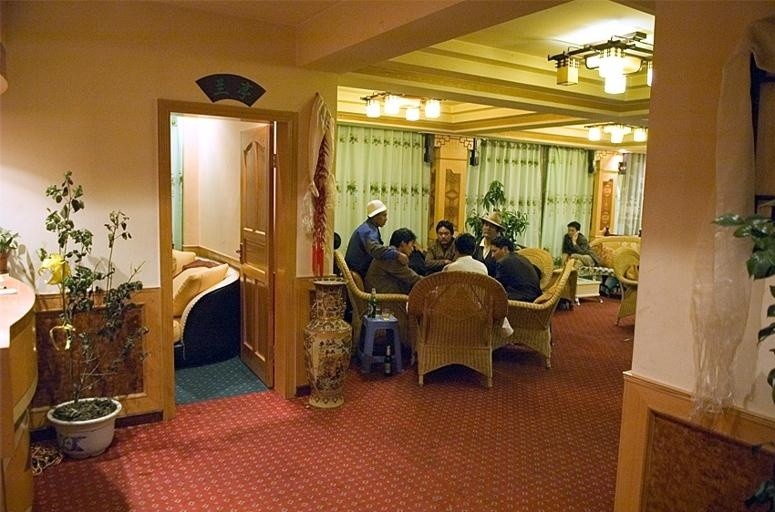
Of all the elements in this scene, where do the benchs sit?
[569,235,641,292]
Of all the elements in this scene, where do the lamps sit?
[584,122,649,144]
[360,91,449,121]
[546,31,655,95]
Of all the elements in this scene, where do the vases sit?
[303,277,353,409]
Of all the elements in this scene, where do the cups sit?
[375,306,395,320]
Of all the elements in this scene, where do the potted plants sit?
[0,226,21,275]
[33,167,152,460]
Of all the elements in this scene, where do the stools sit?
[357,315,403,378]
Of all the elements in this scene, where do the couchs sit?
[170,247,242,368]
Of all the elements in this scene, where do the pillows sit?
[171,248,196,278]
[182,258,221,272]
[171,262,229,319]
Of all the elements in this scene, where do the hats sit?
[477,211,507,233]
[366,198,388,219]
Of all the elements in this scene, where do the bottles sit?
[367,288,377,318]
[384,346,393,375]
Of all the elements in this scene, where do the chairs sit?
[514,248,555,289]
[333,250,409,343]
[493,254,575,369]
[610,246,641,328]
[403,270,513,389]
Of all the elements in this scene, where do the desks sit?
[576,278,605,306]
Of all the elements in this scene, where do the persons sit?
[561,220,599,272]
[344,199,542,338]
